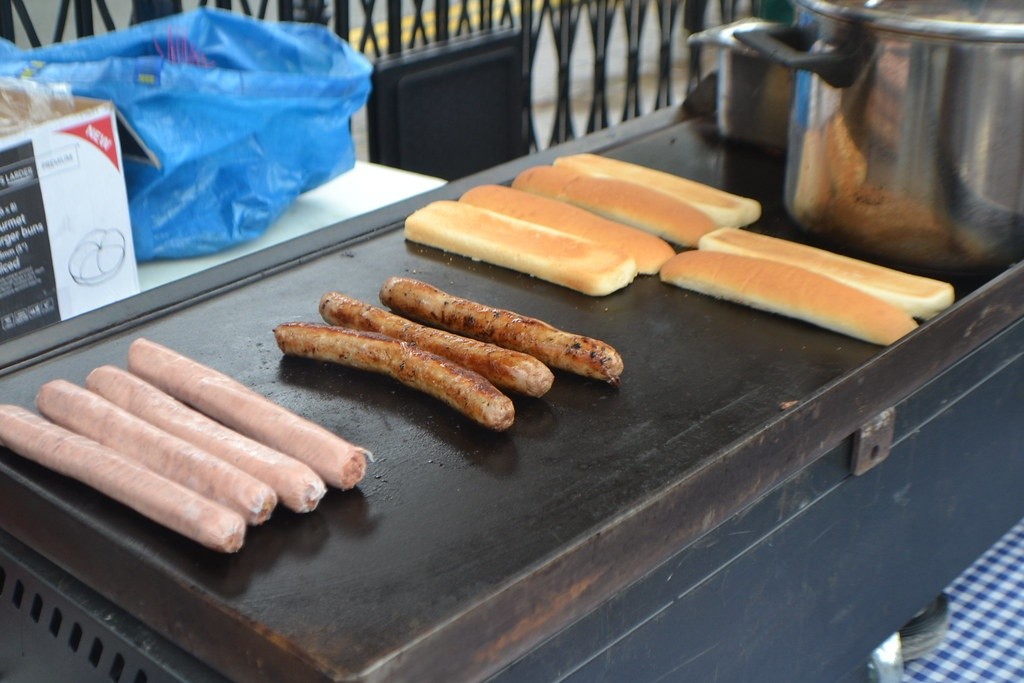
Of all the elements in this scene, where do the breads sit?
[658,227,956,348]
[404,155,762,297]
[787,109,986,267]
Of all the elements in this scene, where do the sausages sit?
[271,276,624,432]
[0,337,368,554]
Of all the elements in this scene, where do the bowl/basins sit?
[687,16,796,152]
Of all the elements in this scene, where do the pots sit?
[735,0,1024,280]
